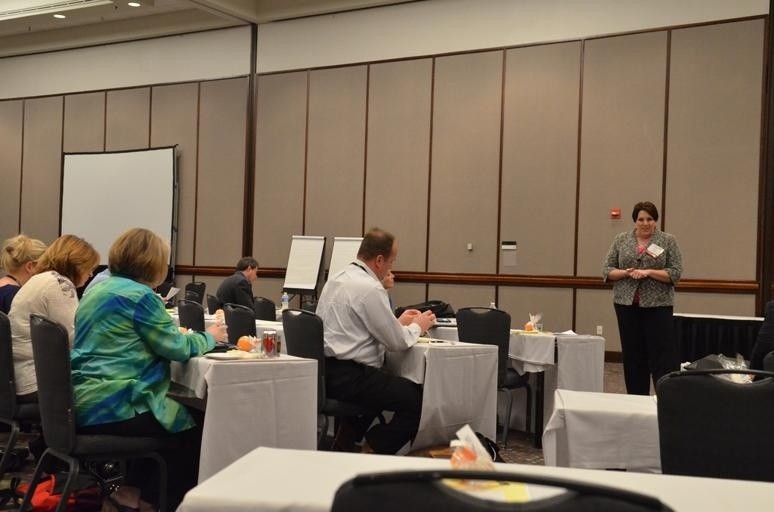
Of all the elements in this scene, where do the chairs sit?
[185,281,205,305]
[302,298,318,313]
[455,306,532,448]
[0,310,41,474]
[330,469,674,512]
[654,367,774,483]
[162,281,177,309]
[206,293,221,315]
[177,298,206,333]
[223,302,257,345]
[19,313,181,512]
[254,296,276,321]
[281,308,386,451]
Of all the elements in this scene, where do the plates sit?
[206,353,240,359]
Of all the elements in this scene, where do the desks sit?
[385,337,499,452]
[541,388,662,475]
[167,353,319,486]
[175,445,774,511]
[424,326,606,448]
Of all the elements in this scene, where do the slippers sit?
[110,485,141,511]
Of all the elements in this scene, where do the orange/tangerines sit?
[238,336,255,352]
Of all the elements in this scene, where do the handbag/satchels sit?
[15,470,103,512]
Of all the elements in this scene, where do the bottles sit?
[261,331,277,357]
[488,303,497,312]
[281,292,288,309]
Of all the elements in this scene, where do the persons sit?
[216,258,258,313]
[0,234,46,315]
[381,269,394,289]
[601,201,684,396]
[70,227,228,512]
[315,228,436,455]
[7,234,101,475]
